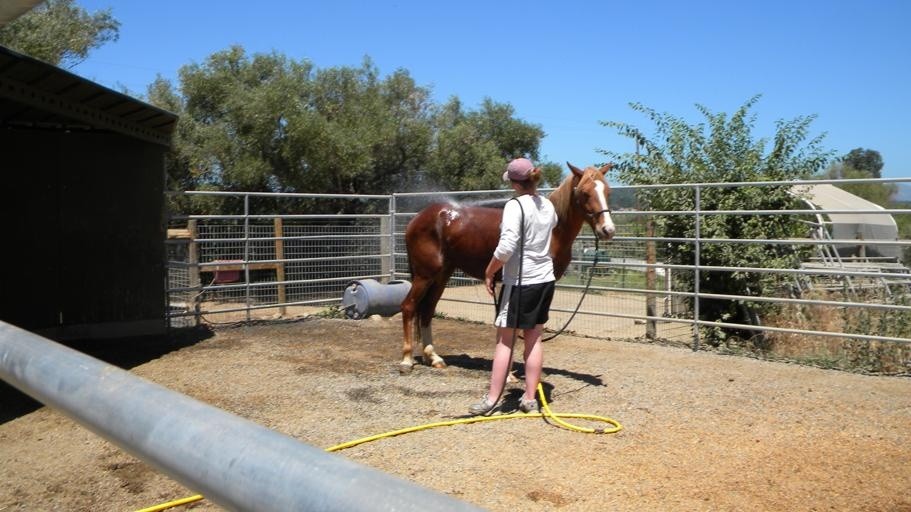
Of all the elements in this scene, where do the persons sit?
[468,157,559,417]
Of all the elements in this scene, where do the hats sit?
[502,157,536,182]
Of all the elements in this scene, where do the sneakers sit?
[469,395,507,416]
[520,395,540,415]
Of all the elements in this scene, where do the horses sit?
[397,160,617,385]
